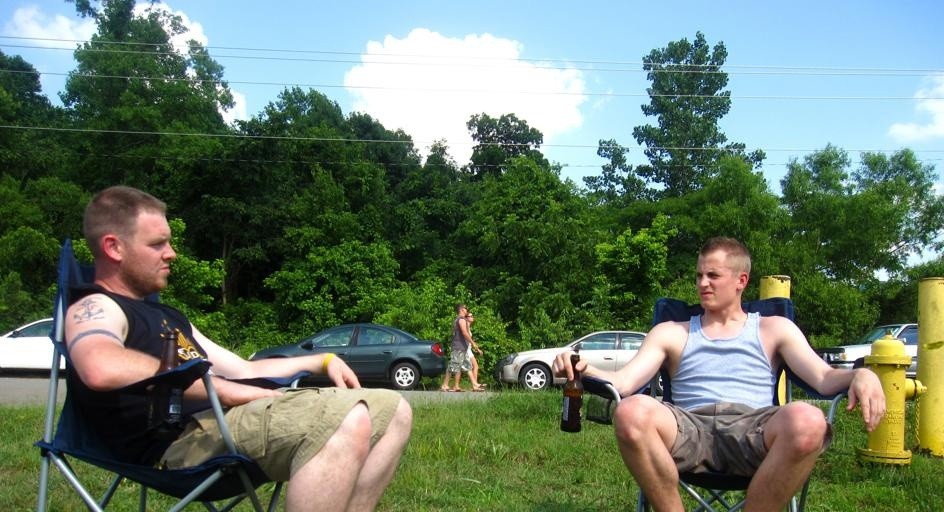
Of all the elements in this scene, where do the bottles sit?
[147,330,183,437]
[560,354,583,433]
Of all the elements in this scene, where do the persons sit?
[452,312,488,392]
[62,184,415,510]
[439,305,488,392]
[549,234,887,511]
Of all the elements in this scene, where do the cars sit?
[0,317,66,369]
[248,323,448,390]
[813,323,918,378]
[493,330,664,396]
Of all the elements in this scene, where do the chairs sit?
[39,240,310,510]
[580,297,849,510]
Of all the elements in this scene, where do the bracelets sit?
[321,352,337,377]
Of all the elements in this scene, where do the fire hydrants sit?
[856,334,927,464]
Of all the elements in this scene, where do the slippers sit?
[438,381,488,392]
